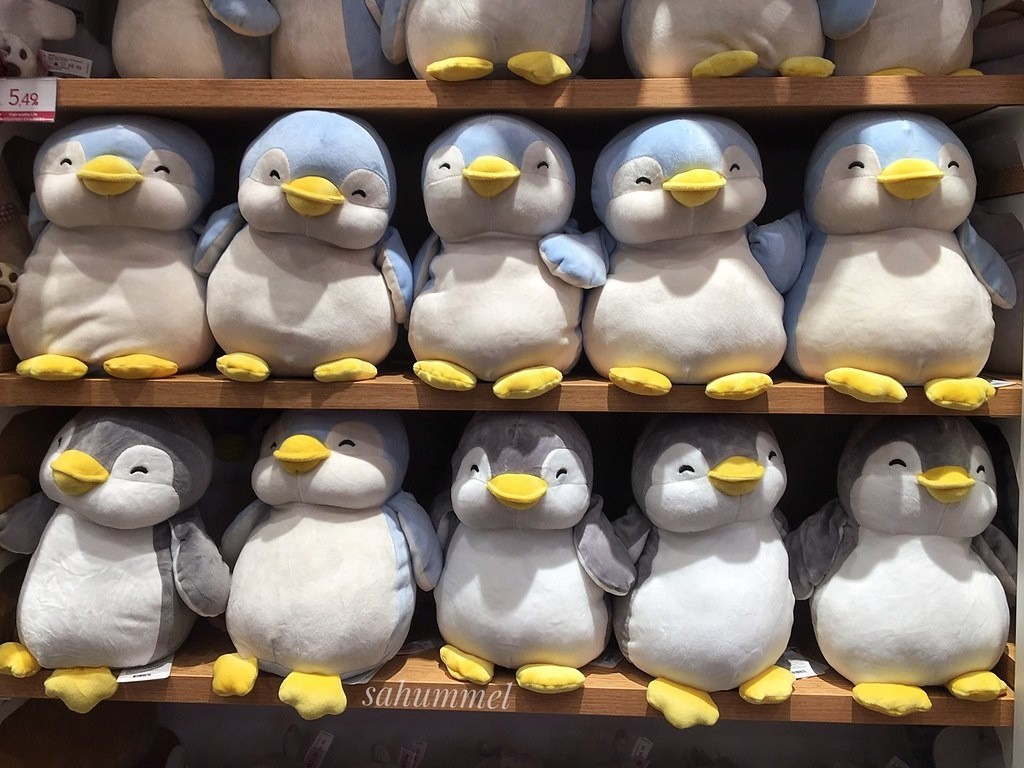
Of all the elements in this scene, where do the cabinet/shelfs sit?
[0,0,1024,768]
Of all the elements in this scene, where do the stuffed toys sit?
[0,0,985,87]
[0,109,1019,401]
[0,408,1018,730]
